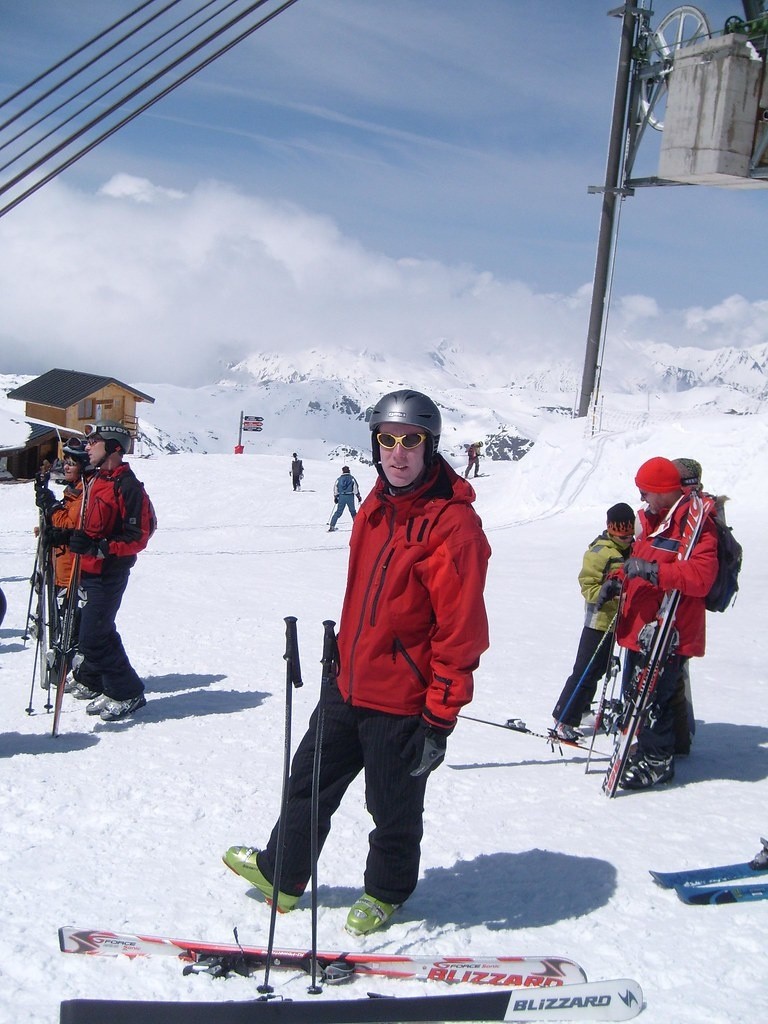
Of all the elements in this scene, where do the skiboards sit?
[599,490,717,799]
[57,924,644,1024]
[647,861,768,906]
[462,473,491,480]
[325,527,339,533]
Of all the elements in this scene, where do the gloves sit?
[34,472,64,519]
[357,496,362,502]
[623,558,658,584]
[334,498,338,504]
[597,580,621,611]
[399,716,455,777]
[45,525,110,559]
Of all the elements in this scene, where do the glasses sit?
[84,423,97,440]
[639,491,646,498]
[63,436,84,451]
[88,437,104,445]
[377,432,427,450]
[63,460,78,467]
[620,533,635,539]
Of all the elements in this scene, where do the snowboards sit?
[455,713,612,757]
[290,488,316,493]
[52,472,89,738]
[34,500,55,690]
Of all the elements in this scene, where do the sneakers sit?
[555,705,688,789]
[222,845,299,914]
[87,692,146,720]
[343,893,403,938]
[62,673,101,699]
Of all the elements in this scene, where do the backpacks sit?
[704,512,743,613]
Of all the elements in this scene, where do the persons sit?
[34,437,102,699]
[50,419,157,722]
[288,453,304,491]
[596,457,729,793]
[551,503,637,740]
[465,441,484,478]
[329,466,362,531]
[220,390,492,936]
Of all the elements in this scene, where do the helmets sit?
[369,389,441,465]
[62,437,90,465]
[87,419,131,454]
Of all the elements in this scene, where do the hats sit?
[635,457,682,493]
[673,458,702,486]
[607,503,635,535]
[342,467,350,473]
